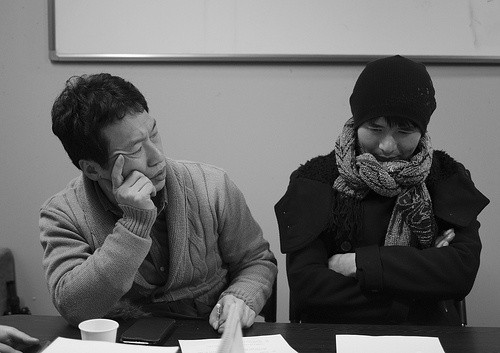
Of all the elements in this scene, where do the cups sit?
[78,319,119,342]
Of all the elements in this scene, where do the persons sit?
[39,73,279,333]
[274,54,490,325]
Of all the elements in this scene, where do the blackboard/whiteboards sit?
[46,0,500,64]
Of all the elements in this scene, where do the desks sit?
[0,315,500,353]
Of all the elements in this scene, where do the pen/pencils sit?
[122,161,132,176]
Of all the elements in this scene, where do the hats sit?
[350,55,437,136]
